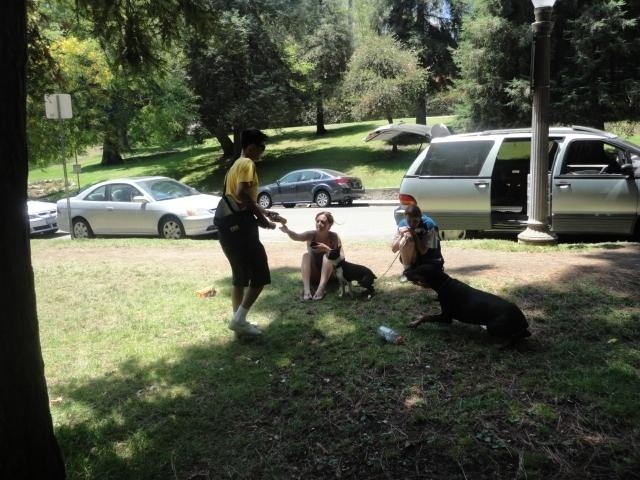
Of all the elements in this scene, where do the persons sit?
[391,205,444,282]
[279,211,345,300]
[214,127,287,340]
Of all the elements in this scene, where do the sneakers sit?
[228,320,261,341]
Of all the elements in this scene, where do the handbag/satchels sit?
[215,195,248,235]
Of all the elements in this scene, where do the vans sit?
[361,123,640,241]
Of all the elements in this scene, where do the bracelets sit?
[265,210,270,217]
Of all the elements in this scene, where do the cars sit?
[26,198,58,235]
[256,168,365,209]
[184,117,235,139]
[56,176,222,240]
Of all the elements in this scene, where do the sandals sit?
[303,292,311,300]
[314,290,325,300]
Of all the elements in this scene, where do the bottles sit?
[265,212,288,226]
[377,325,405,344]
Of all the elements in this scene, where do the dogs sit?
[403,261,532,350]
[325,243,377,300]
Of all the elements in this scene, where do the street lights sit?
[517,0,558,247]
